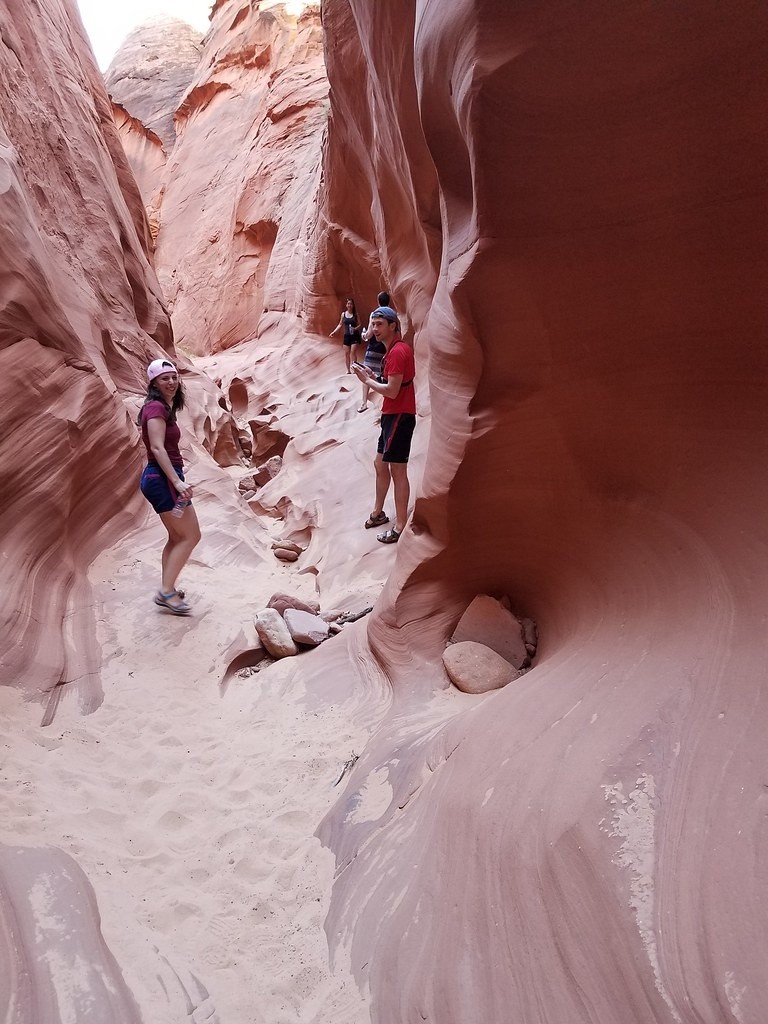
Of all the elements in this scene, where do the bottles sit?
[171,494,190,519]
[349,324,354,335]
[362,327,369,342]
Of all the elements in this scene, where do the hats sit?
[372,307,398,323]
[147,359,177,381]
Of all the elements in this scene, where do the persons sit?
[138,359,201,612]
[329,298,362,374]
[351,307,416,544]
[357,291,400,412]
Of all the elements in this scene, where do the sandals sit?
[155,591,191,613]
[376,527,401,544]
[175,590,185,599]
[365,512,389,529]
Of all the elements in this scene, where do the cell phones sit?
[353,361,366,369]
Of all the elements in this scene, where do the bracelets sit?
[363,335,366,340]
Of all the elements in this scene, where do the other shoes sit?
[357,403,368,412]
